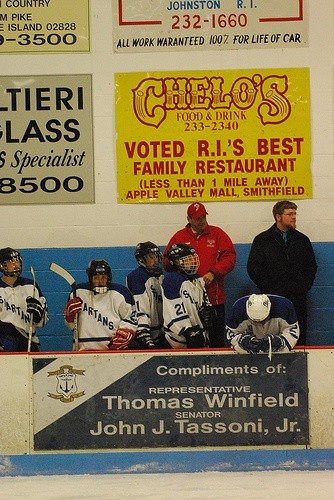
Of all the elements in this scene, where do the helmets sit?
[166,243,200,275]
[135,241,163,273]
[0,247,24,277]
[86,259,112,294]
[246,294,271,322]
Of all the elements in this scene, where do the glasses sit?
[281,212,297,216]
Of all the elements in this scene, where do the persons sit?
[226,294,300,354]
[163,201,236,347]
[64,259,138,351]
[160,243,218,349]
[0,247,49,351]
[247,201,318,345]
[126,241,165,350]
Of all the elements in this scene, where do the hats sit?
[187,202,208,220]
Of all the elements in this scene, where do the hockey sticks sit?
[49,262,80,351]
[28,265,36,351]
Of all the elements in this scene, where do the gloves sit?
[260,333,285,353]
[198,306,217,329]
[108,328,136,350]
[26,296,44,323]
[238,333,263,355]
[142,336,157,349]
[183,326,206,348]
[64,297,83,323]
